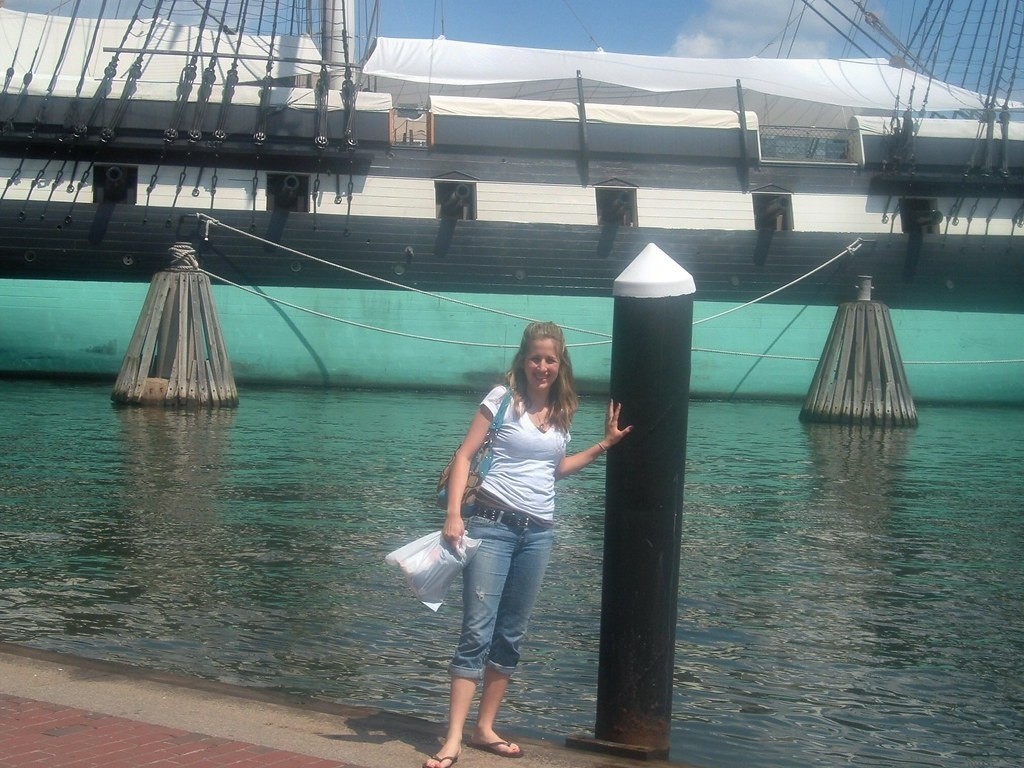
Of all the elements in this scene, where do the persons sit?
[425,321,636,768]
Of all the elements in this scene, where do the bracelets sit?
[598,443,606,451]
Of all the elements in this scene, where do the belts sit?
[471,503,539,528]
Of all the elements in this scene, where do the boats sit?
[0,0,1024,403]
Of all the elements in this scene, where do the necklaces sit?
[531,403,552,431]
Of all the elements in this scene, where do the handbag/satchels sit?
[436,444,494,517]
[384,524,483,612]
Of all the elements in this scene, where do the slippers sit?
[422,754,457,768]
[467,732,523,758]
